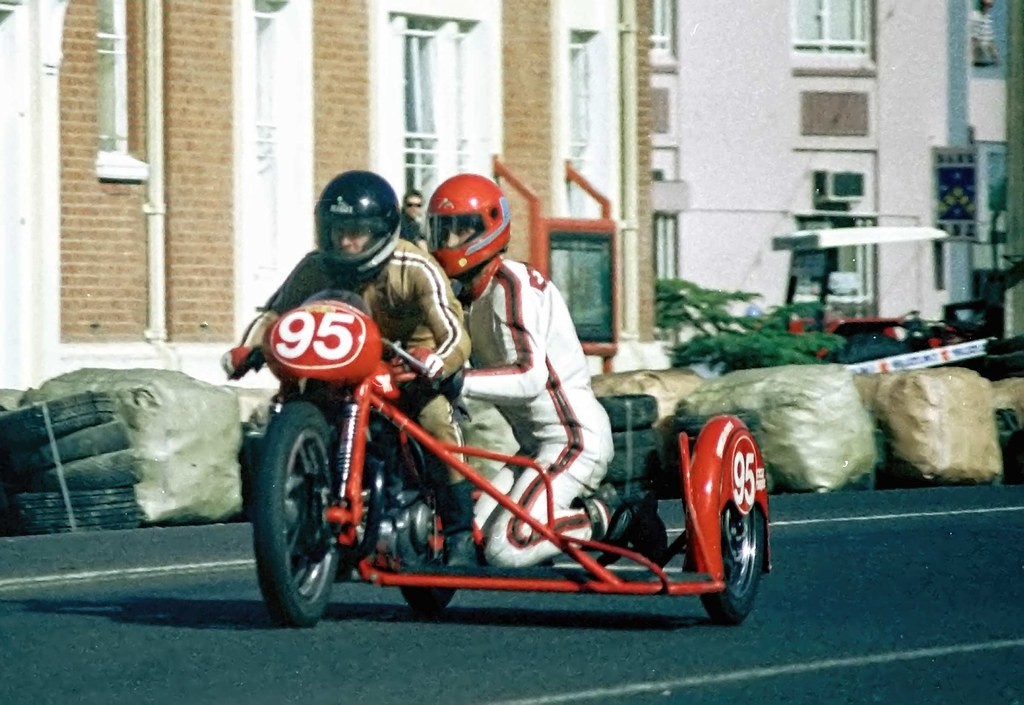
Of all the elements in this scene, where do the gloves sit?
[401,345,445,382]
[220,346,254,380]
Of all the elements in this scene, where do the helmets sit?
[314,169,401,275]
[426,172,512,278]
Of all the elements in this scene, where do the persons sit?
[400,189,427,243]
[390,174,670,569]
[221,171,476,568]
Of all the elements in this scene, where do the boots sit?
[570,473,667,570]
[434,476,478,568]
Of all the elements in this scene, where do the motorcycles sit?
[226,290,772,628]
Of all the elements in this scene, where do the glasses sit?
[403,199,423,207]
